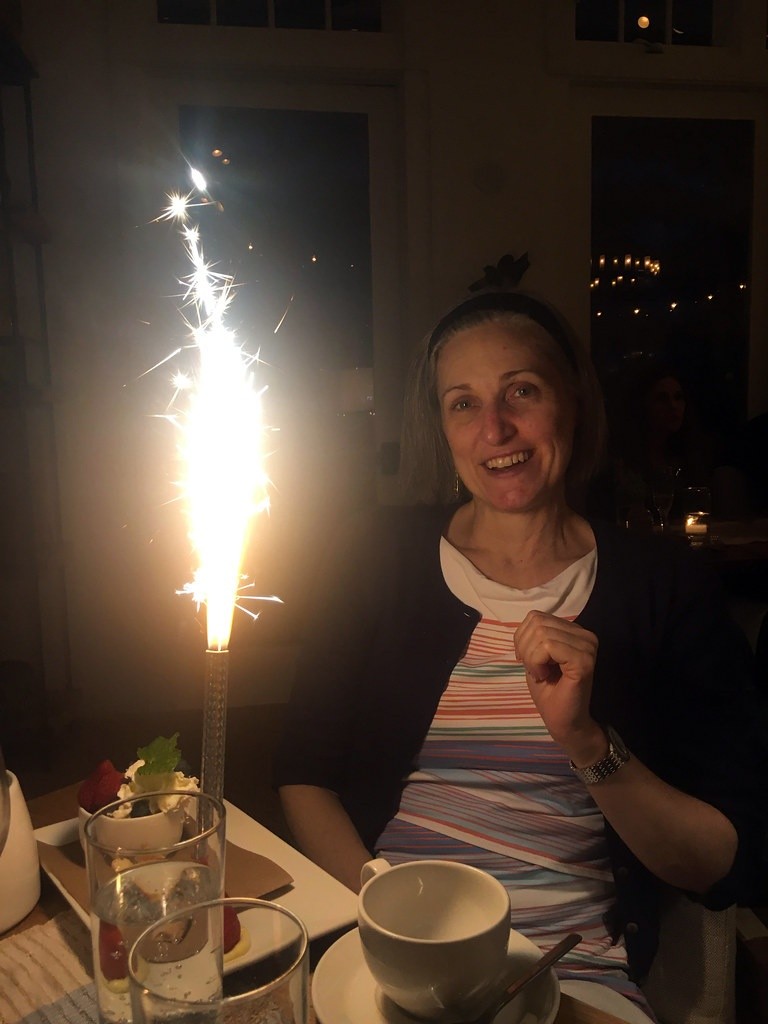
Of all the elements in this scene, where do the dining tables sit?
[1,771,629,1024]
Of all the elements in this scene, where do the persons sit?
[270,291,768,1020]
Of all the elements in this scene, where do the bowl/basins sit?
[76,780,188,862]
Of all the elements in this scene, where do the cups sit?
[126,896,311,1024]
[83,790,226,1024]
[358,858,510,1016]
[684,486,714,541]
[0,765,41,937]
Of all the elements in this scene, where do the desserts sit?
[73,737,205,821]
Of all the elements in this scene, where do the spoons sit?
[409,930,585,1024]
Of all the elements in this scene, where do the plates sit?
[311,923,561,1024]
[32,792,365,976]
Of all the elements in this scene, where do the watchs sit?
[569,727,633,786]
